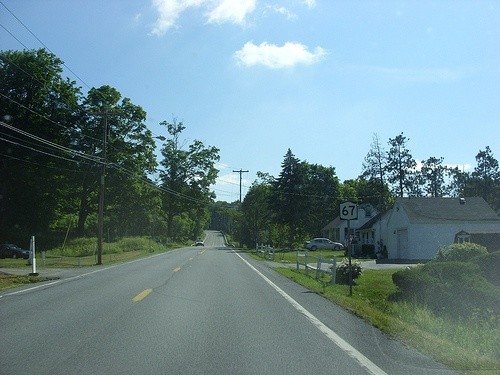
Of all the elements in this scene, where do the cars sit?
[0,243,29,259]
[195,239,204,246]
[305,237,345,251]
